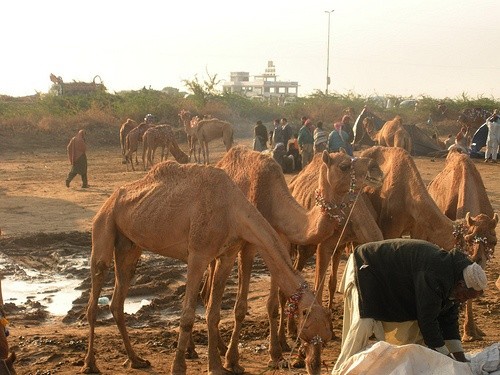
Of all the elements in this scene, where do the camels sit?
[120,111,234,171]
[362,115,412,154]
[80,144,499,375]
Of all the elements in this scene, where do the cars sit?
[284,97,296,106]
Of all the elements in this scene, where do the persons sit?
[298,116,315,170]
[281,118,292,145]
[253,120,268,151]
[270,119,283,151]
[340,115,355,158]
[332,238,488,375]
[452,126,475,155]
[313,122,330,154]
[273,142,303,173]
[484,112,500,163]
[327,121,350,154]
[65,130,90,189]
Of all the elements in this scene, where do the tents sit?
[470,109,500,158]
[352,103,445,158]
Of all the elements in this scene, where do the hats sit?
[463,262,487,291]
